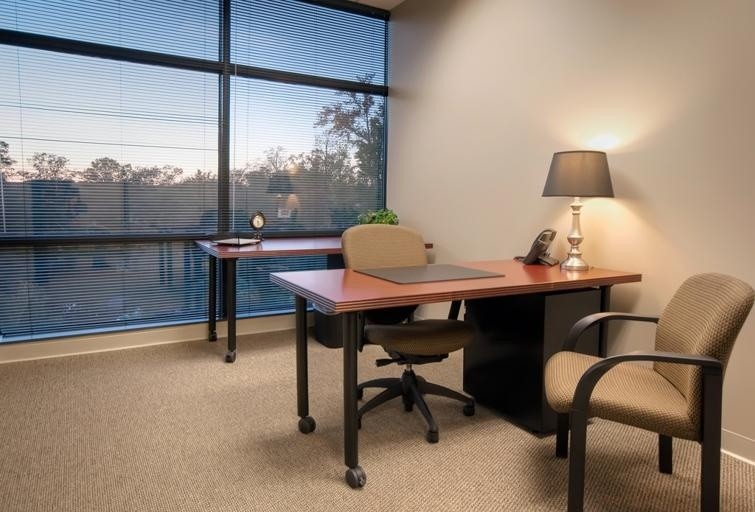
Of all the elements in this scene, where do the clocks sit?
[250,209,266,241]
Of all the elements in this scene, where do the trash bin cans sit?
[257,265,289,309]
[312,302,343,348]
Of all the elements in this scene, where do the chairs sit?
[544,273,755,512]
[341,224,475,443]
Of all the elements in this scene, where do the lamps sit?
[542,150,615,271]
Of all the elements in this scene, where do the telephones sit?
[523,229,559,266]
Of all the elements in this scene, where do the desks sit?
[270,259,642,488]
[196,237,433,363]
